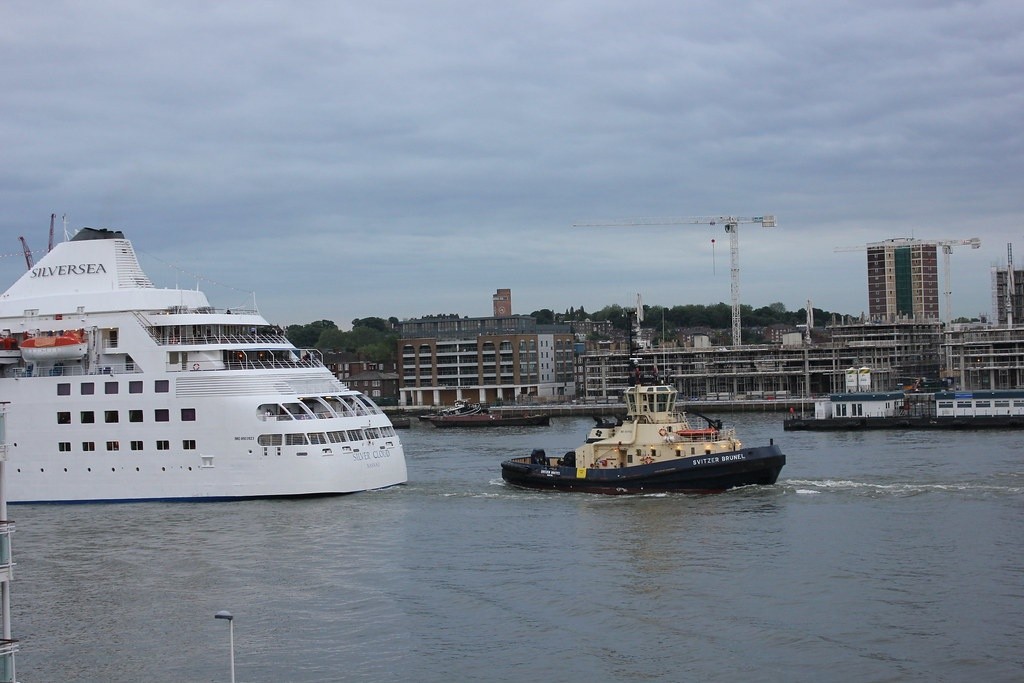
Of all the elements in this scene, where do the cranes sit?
[571,213,777,344]
[835,228,980,378]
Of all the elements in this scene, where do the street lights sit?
[214,609,236,683]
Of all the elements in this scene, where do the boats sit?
[384,407,410,428]
[780,389,1024,431]
[0,211,410,506]
[499,309,787,493]
[0,337,22,364]
[419,398,551,427]
[18,336,87,363]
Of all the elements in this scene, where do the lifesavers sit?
[659,428,667,436]
[193,363,200,370]
